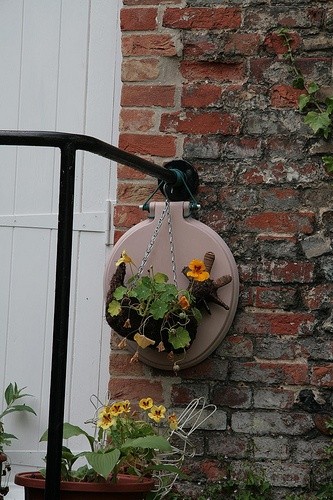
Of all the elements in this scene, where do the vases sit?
[14,470,156,500]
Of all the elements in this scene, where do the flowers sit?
[38,397,192,485]
[107,248,211,378]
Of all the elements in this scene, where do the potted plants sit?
[0,382,38,500]
